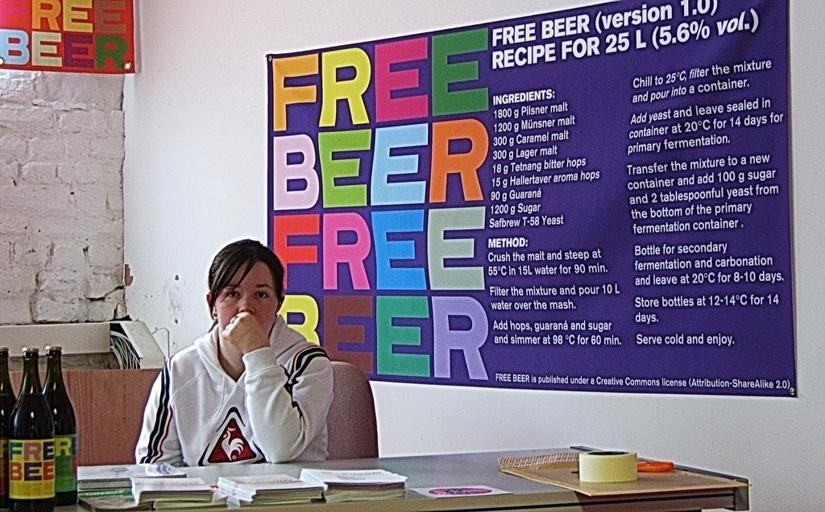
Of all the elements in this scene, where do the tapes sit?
[578,450,638,483]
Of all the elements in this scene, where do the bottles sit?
[0,343,80,512]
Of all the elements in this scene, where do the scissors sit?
[570,461,675,473]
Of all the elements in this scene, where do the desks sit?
[0,449,752,512]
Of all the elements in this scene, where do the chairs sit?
[329,361,379,466]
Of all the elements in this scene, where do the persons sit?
[134,239,332,466]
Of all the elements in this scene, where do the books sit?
[76,464,407,511]
